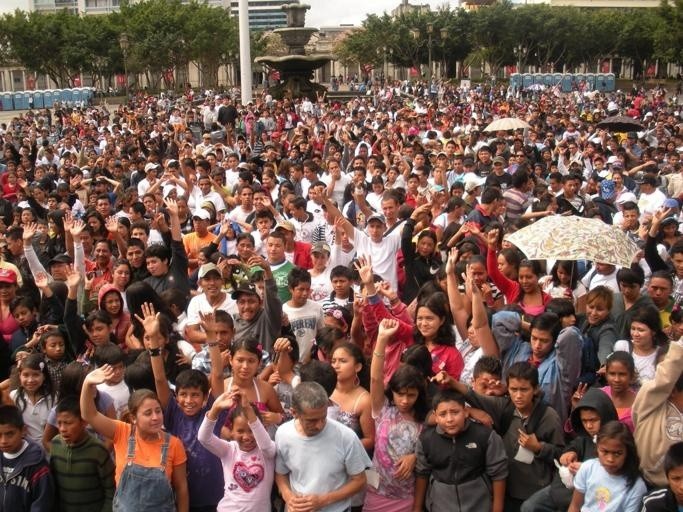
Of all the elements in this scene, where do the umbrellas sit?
[482,118,531,133]
[595,115,644,137]
[507,214,643,296]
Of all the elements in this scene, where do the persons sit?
[493,310,583,418]
[568,420,648,512]
[135,302,228,511]
[229,235,512,339]
[642,207,682,306]
[431,361,564,512]
[197,386,277,512]
[273,334,343,421]
[0,404,57,511]
[487,230,552,315]
[607,263,662,339]
[469,355,507,398]
[328,342,378,454]
[539,260,586,297]
[1,239,235,364]
[361,319,428,511]
[273,380,371,511]
[519,388,619,511]
[358,67,683,232]
[564,350,637,433]
[51,396,115,511]
[640,442,683,512]
[220,338,281,412]
[80,364,188,512]
[647,273,678,336]
[579,262,621,296]
[0,354,59,463]
[2,69,357,238]
[412,389,508,511]
[546,298,599,372]
[572,286,614,366]
[631,336,683,489]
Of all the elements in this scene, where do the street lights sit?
[119,33,130,98]
[377,46,393,79]
[222,50,239,86]
[413,23,448,89]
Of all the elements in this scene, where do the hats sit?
[367,213,386,224]
[275,221,296,236]
[494,156,506,165]
[0,269,17,285]
[310,241,331,256]
[600,179,616,200]
[198,262,224,281]
[48,254,74,263]
[464,158,474,166]
[91,177,107,184]
[635,172,656,186]
[144,163,161,172]
[659,198,679,209]
[430,185,446,195]
[465,177,486,192]
[232,283,263,304]
[191,209,210,221]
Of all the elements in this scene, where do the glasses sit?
[516,155,524,157]
